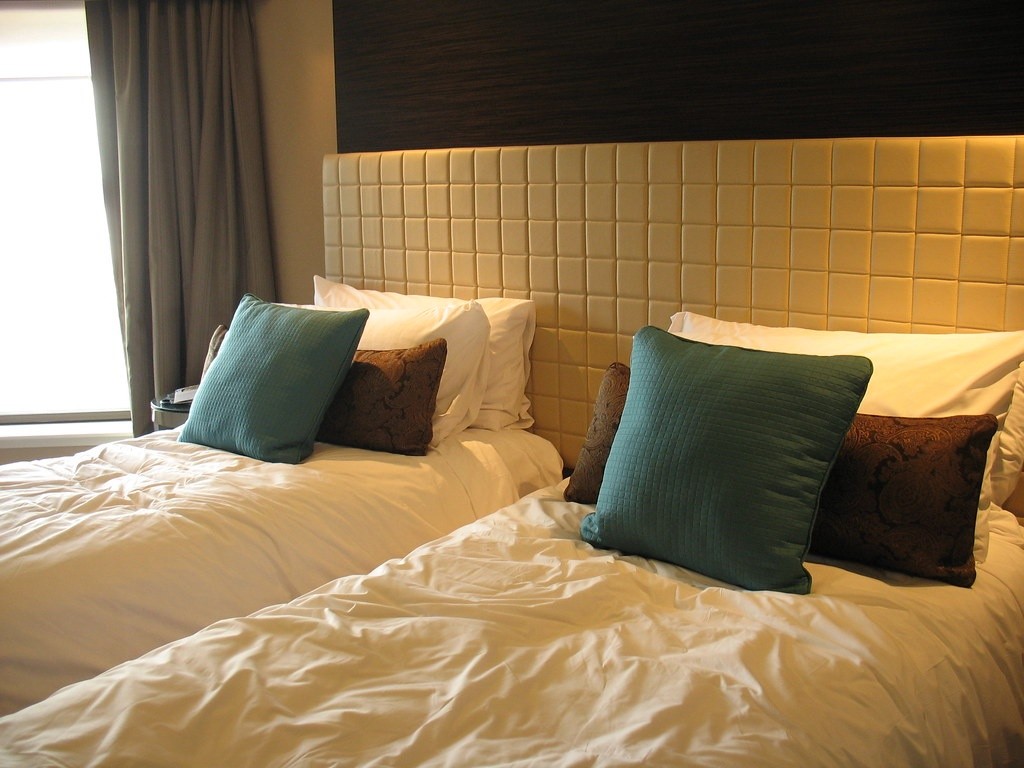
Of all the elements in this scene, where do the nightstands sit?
[151,394,190,432]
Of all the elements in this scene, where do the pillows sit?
[313,274,537,431]
[270,300,491,451]
[809,414,998,588]
[987,362,1024,551]
[564,362,630,504]
[668,311,1024,563]
[177,292,370,465]
[314,337,447,455]
[201,324,228,384]
[578,325,874,595]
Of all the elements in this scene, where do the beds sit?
[0,477,1024,768]
[0,426,561,717]
